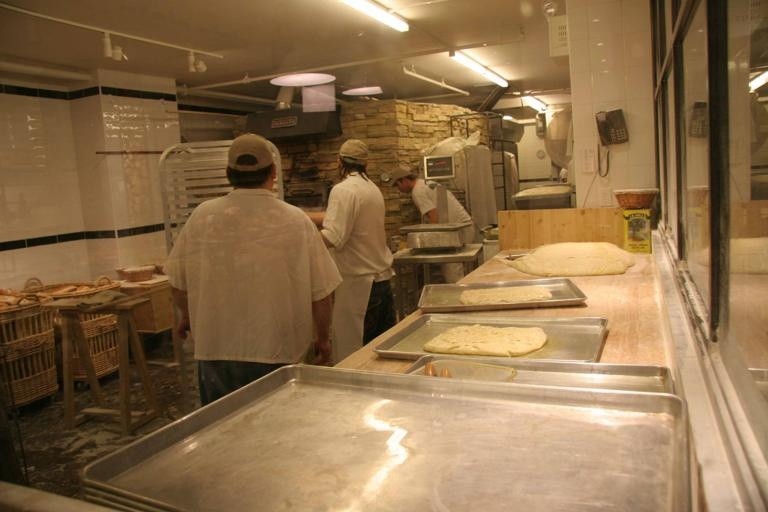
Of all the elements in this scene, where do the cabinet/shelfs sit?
[155,141,284,275]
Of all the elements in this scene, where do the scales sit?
[400,154,471,253]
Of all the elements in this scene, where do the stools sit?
[47,297,163,436]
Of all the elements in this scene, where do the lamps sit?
[95,32,124,62]
[186,49,207,74]
[748,70,768,92]
[271,72,337,87]
[524,95,546,111]
[341,86,382,96]
[449,51,509,88]
[344,0,409,33]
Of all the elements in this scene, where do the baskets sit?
[0,275,121,409]
[613,189,657,209]
[123,266,155,282]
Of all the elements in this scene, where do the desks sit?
[390,243,483,323]
[511,185,572,210]
[119,274,181,370]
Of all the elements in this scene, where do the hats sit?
[390,169,409,186]
[228,133,272,171]
[339,139,369,160]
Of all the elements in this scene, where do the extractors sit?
[242,102,343,140]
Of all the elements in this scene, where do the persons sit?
[309,137,398,369]
[162,131,344,413]
[386,167,479,283]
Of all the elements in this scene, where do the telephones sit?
[595,108,629,146]
